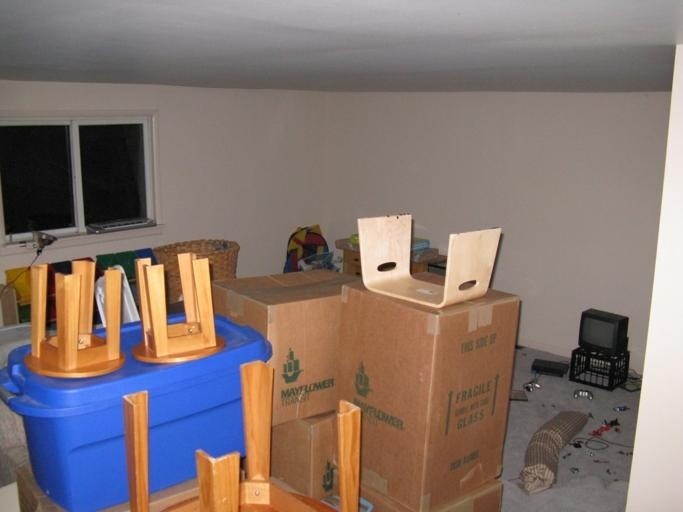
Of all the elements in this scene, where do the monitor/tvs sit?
[579,308,628,355]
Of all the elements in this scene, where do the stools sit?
[356,214,502,309]
[24,260,127,378]
[132,253,225,365]
[121,360,362,512]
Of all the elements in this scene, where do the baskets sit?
[152,240,240,305]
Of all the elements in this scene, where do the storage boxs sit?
[270,419,340,499]
[1,312,272,511]
[209,270,359,426]
[339,271,521,511]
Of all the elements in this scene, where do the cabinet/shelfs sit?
[336,235,447,284]
